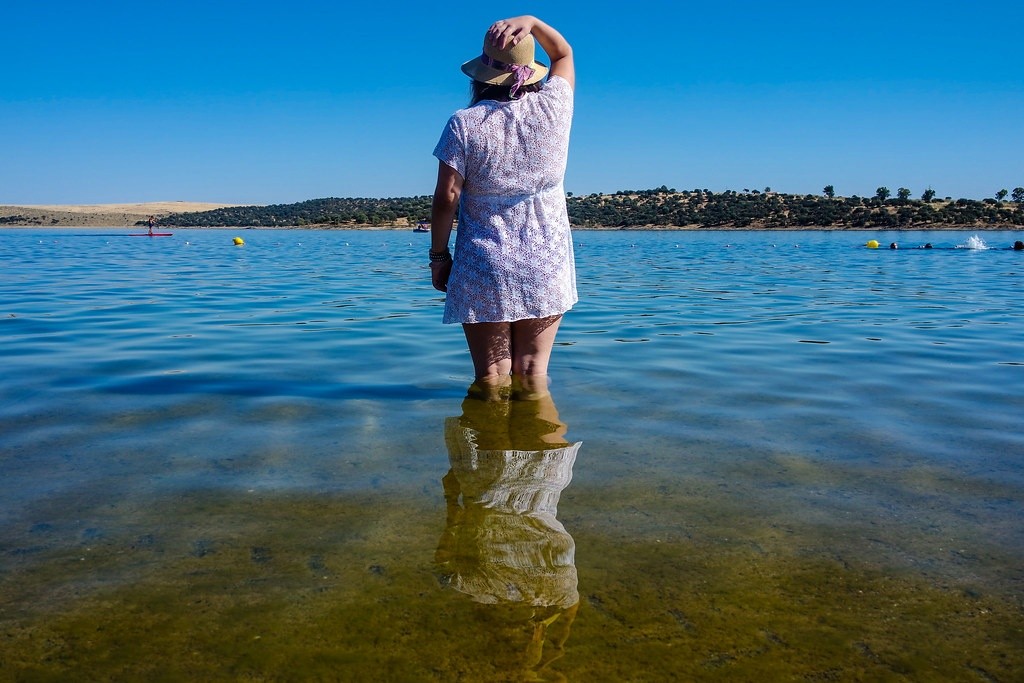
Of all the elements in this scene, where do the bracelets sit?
[429,247,450,262]
[445,493,458,499]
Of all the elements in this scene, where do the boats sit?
[128,233,173,236]
[413,229,429,233]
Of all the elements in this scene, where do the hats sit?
[460,29,550,99]
[477,610,560,668]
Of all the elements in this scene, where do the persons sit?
[1014,241,1024,250]
[428,15,579,375]
[435,375,584,666]
[148,216,155,234]
[418,225,421,229]
[890,243,897,249]
[424,225,427,229]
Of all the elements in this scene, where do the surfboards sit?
[128,233,173,236]
